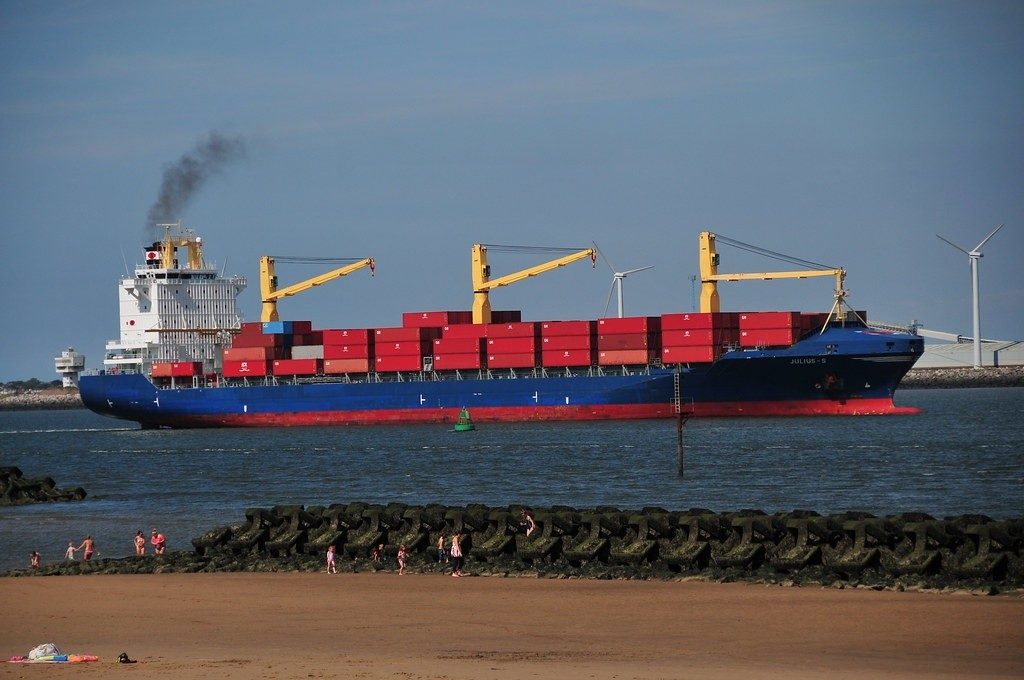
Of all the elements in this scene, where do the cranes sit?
[470,243,599,325]
[255,255,376,322]
[697,227,851,325]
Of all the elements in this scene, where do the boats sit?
[453,407,477,432]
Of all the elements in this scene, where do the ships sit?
[74,231,929,417]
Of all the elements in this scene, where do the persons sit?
[450,530,465,578]
[519,509,536,537]
[151,528,166,555]
[437,531,450,564]
[30,551,42,566]
[373,544,386,563]
[134,531,146,555]
[326,545,337,574]
[397,545,410,576]
[64,542,79,559]
[75,534,100,560]
